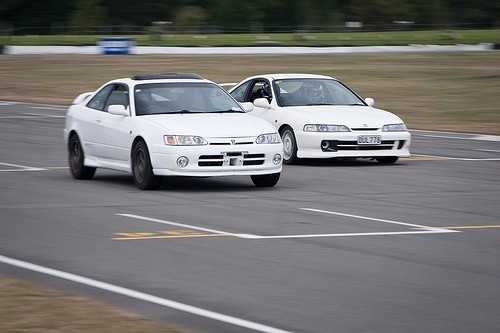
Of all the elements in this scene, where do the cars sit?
[227,73,411,164]
[64,72,283,186]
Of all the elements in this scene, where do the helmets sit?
[303,82,323,104]
[260,82,281,102]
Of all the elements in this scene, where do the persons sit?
[135,87,158,113]
[262,82,285,106]
[301,82,324,103]
